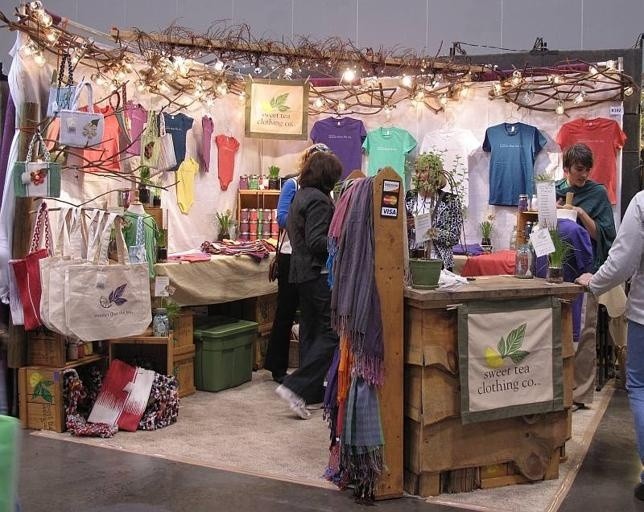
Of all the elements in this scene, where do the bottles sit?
[515,245,536,279]
[238,175,257,189]
[240,208,279,240]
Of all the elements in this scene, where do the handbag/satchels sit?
[13,160,62,198]
[47,85,78,117]
[158,134,177,171]
[8,243,153,341]
[268,256,279,282]
[140,135,161,169]
[59,111,104,149]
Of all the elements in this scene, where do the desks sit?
[153,247,282,399]
[403,276,590,500]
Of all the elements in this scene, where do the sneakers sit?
[275,383,312,420]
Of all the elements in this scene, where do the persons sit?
[264,142,337,385]
[575,147,644,501]
[272,150,343,420]
[553,142,617,413]
[405,156,461,273]
[555,209,594,353]
[120,202,165,282]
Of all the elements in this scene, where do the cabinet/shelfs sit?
[237,190,280,242]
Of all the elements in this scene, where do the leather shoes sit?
[272,372,289,384]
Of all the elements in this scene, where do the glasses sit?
[310,144,328,152]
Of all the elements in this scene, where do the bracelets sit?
[587,280,590,293]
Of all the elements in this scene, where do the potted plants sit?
[478,215,495,250]
[407,147,469,289]
[541,227,582,283]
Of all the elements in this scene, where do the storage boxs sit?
[16,332,107,435]
[192,318,258,394]
